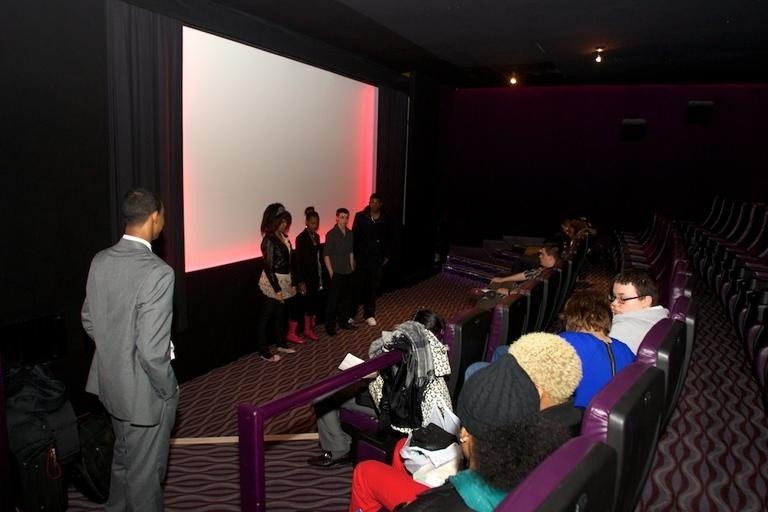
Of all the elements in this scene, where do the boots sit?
[303,315,320,340]
[286,319,305,343]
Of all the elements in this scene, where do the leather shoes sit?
[278,346,295,354]
[260,354,281,361]
[306,454,336,465]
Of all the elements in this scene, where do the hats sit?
[458,354,540,437]
[508,332,582,398]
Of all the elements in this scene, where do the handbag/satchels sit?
[377,382,423,428]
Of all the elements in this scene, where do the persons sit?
[464,292,636,407]
[307,309,447,466]
[606,270,671,356]
[348,194,392,326]
[508,331,583,411]
[560,220,571,236]
[489,240,561,297]
[495,220,589,279]
[257,203,296,363]
[80,188,180,511]
[287,207,321,344]
[322,209,359,336]
[349,353,569,511]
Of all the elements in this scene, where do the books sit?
[337,352,377,379]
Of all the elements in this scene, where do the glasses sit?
[607,293,643,304]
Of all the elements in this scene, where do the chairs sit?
[335,190,767,512]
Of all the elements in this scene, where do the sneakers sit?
[328,317,377,336]
